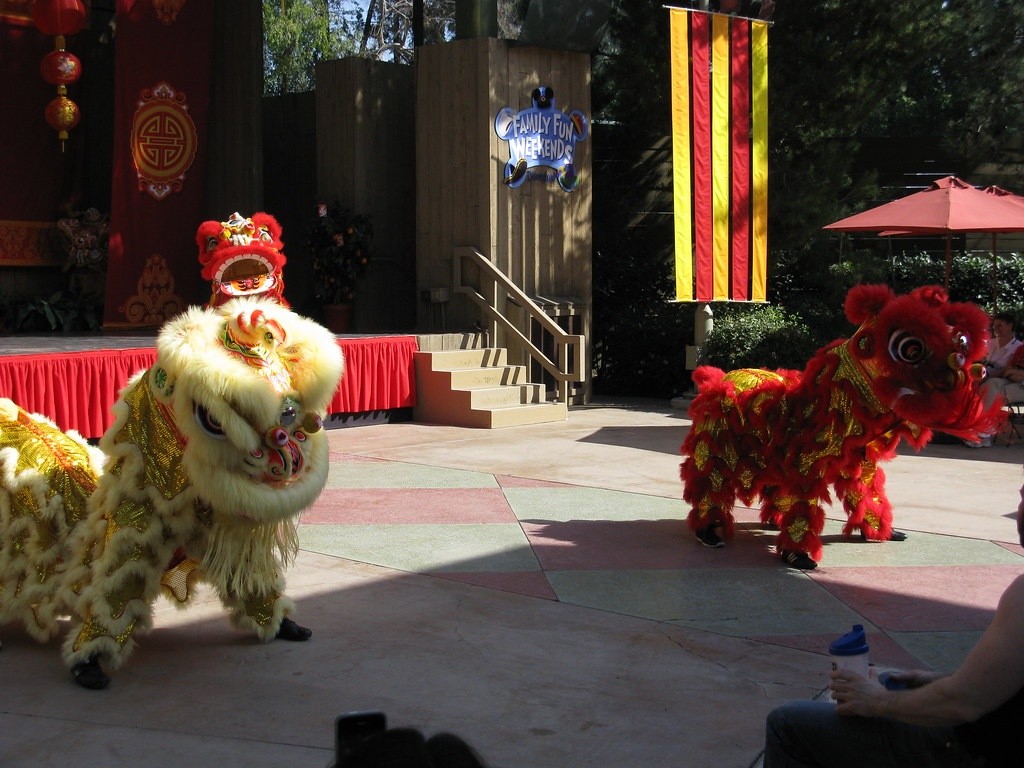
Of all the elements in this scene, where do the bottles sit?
[828,623,870,698]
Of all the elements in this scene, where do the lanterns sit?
[41,49,81,95]
[45,97,79,152]
[33,0,87,45]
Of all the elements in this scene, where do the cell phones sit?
[334,710,387,768]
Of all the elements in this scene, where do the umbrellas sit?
[821,176,1024,320]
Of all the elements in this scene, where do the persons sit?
[962,313,1024,447]
[764,485,1024,768]
[329,726,482,768]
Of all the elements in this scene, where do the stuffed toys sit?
[0,297,344,689]
[680,284,1005,570]
[196,212,291,309]
[60,209,110,271]
